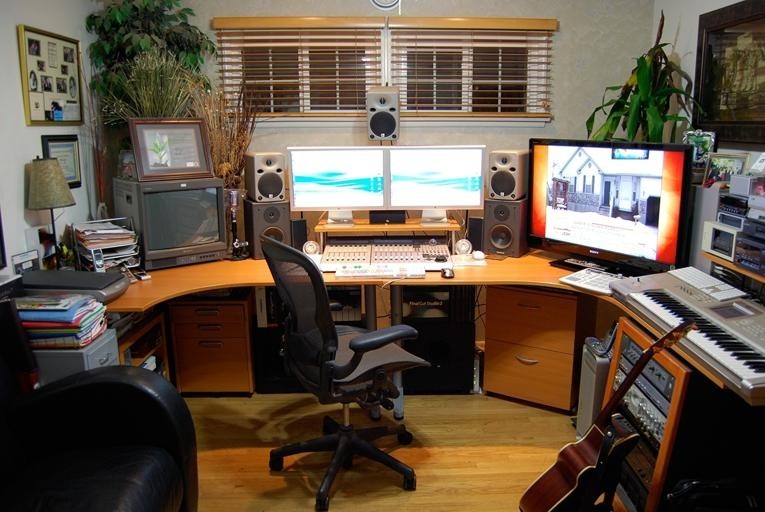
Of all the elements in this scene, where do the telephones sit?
[92,247,107,274]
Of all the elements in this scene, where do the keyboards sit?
[559,266,630,296]
[335,263,425,279]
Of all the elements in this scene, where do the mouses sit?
[441,267,454,278]
[472,250,485,260]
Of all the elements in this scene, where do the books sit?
[14,294,109,349]
[66,224,135,249]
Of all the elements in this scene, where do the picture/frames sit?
[17,23,86,127]
[129,115,216,181]
[701,153,751,189]
[680,131,714,184]
[686,2,765,145]
[41,134,82,190]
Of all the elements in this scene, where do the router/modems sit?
[473,354,479,392]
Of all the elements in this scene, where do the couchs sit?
[261,235,433,512]
[1,298,199,512]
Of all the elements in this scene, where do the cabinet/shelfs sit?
[483,285,598,414]
[168,289,257,398]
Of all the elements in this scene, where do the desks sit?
[36,214,765,512]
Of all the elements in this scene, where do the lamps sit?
[24,155,75,264]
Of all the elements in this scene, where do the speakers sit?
[467,217,483,250]
[242,151,285,202]
[303,241,319,256]
[289,219,307,251]
[367,84,400,141]
[481,198,530,258]
[489,149,530,200]
[243,199,291,260]
[454,239,472,255]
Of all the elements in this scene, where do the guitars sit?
[519,316,701,512]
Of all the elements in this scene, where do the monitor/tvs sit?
[525,138,694,277]
[287,147,386,228]
[386,145,486,227]
[112,176,228,271]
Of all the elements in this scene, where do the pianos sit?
[608,266,765,407]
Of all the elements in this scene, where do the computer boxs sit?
[575,345,613,441]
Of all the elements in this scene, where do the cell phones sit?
[91,248,105,272]
[134,271,151,280]
